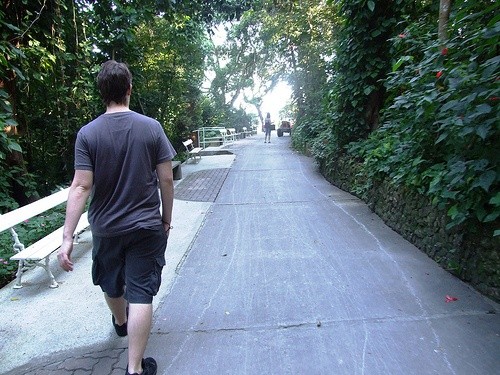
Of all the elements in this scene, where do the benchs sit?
[182,138,203,164]
[220,127,252,143]
[0,186,90,289]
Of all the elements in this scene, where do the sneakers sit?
[112,303,128,336]
[125,357,157,375]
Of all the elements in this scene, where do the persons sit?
[264,113,271,143]
[57,61,177,375]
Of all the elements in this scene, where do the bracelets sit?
[161,219,172,233]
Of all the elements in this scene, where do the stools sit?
[171,161,181,180]
